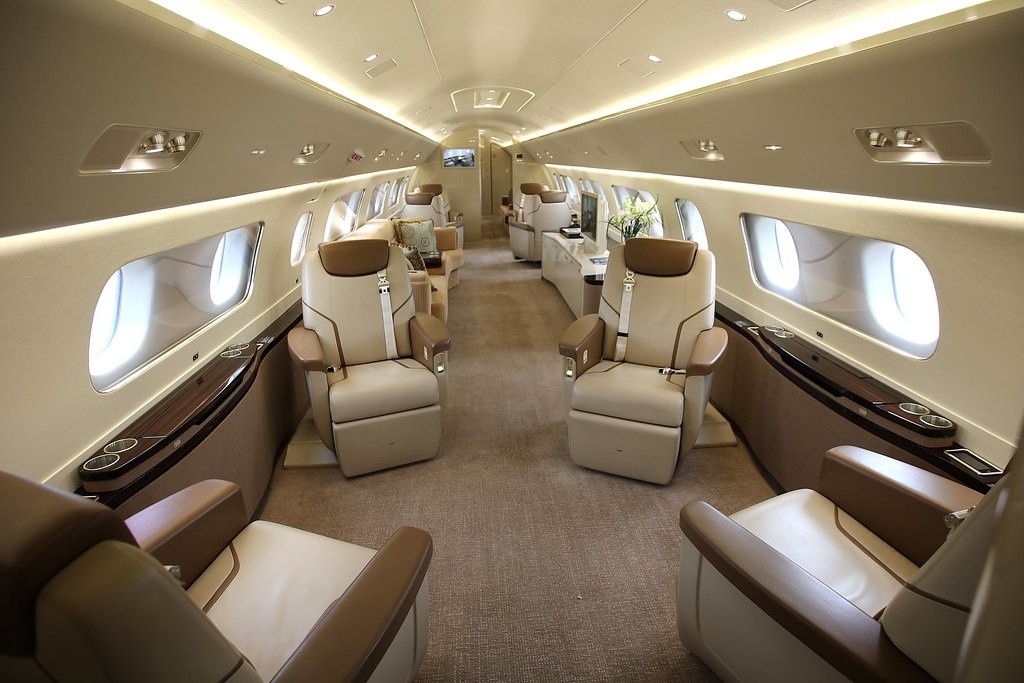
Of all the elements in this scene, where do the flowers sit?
[604,192,660,243]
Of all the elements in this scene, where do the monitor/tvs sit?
[580,190,607,254]
[443,147,476,168]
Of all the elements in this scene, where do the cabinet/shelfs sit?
[540,230,610,318]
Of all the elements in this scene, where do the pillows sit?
[392,215,423,243]
[397,219,437,252]
[387,241,439,292]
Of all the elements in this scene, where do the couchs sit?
[333,218,464,325]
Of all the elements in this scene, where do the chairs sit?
[413,183,463,224]
[399,192,464,250]
[674,418,1023,683]
[506,190,572,262]
[281,239,453,479]
[555,234,731,488]
[504,183,549,237]
[0,468,436,683]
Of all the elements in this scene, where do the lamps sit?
[142,131,163,153]
[868,129,887,147]
[894,127,921,147]
[302,145,313,155]
[167,135,184,153]
[699,140,708,151]
[708,141,716,150]
